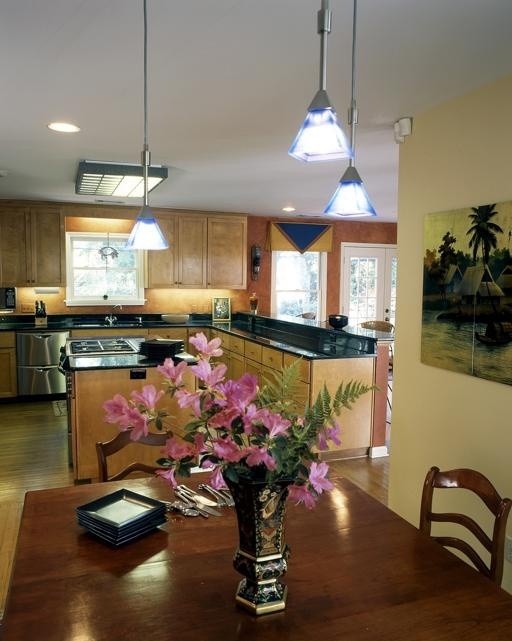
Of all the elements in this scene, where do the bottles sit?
[249,293,259,315]
[35,300,47,326]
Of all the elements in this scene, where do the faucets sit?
[110,303,122,324]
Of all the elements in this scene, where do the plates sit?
[74,489,167,547]
[138,339,184,357]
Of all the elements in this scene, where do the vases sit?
[229,481,296,616]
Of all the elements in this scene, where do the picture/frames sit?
[212,297,231,321]
[212,322,231,331]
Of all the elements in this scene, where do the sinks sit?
[72,321,145,328]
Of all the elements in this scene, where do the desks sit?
[0,468,512,641]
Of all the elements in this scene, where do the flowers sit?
[101,332,384,513]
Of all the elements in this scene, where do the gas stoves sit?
[57,336,198,371]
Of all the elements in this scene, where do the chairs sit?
[419,466,512,589]
[354,321,395,367]
[95,426,173,483]
[297,312,317,319]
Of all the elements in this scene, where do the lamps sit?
[287,0,354,164]
[125,0,169,251]
[75,161,169,198]
[323,0,377,220]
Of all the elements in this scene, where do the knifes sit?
[174,483,235,518]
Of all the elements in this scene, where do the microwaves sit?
[0,287,17,311]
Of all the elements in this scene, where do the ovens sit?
[16,328,71,398]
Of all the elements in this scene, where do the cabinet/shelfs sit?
[0,204,66,287]
[145,215,208,288]
[1,329,18,400]
[207,213,248,289]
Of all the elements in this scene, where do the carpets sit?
[51,400,67,417]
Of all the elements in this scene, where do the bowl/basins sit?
[161,315,190,324]
[329,315,348,328]
